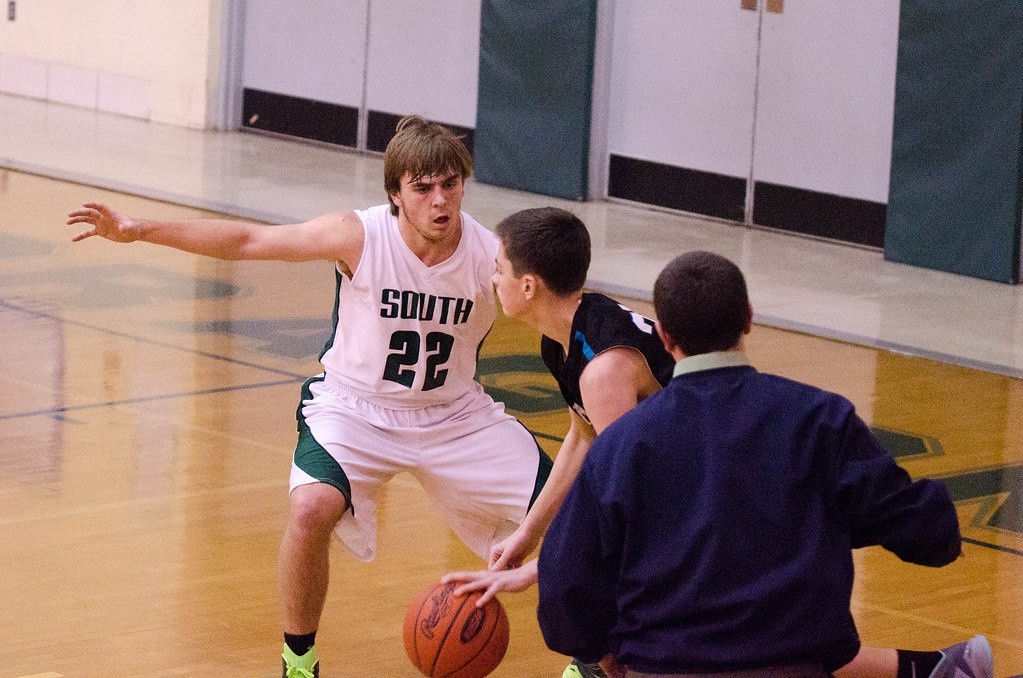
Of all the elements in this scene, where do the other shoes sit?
[929,635,995,678]
[561,653,606,678]
[282,643,319,678]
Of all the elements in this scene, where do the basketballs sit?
[403,581,510,678]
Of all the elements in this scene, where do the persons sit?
[66,114,555,678]
[442,205,993,678]
[536,250,962,678]
